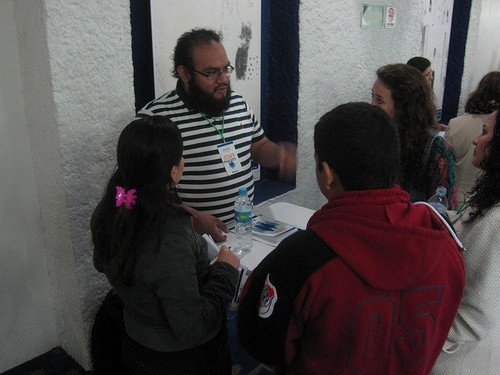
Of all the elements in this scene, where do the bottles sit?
[234,188,252,252]
[427,186,448,216]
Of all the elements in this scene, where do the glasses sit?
[193,65,234,80]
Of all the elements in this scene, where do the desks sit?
[214,188,328,277]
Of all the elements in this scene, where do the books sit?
[235,212,298,243]
[200,234,244,304]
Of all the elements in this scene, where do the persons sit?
[369,64,458,212]
[134,28,295,244]
[429,109,500,375]
[407,57,448,132]
[444,70,500,213]
[89,115,240,375]
[237,102,466,375]
